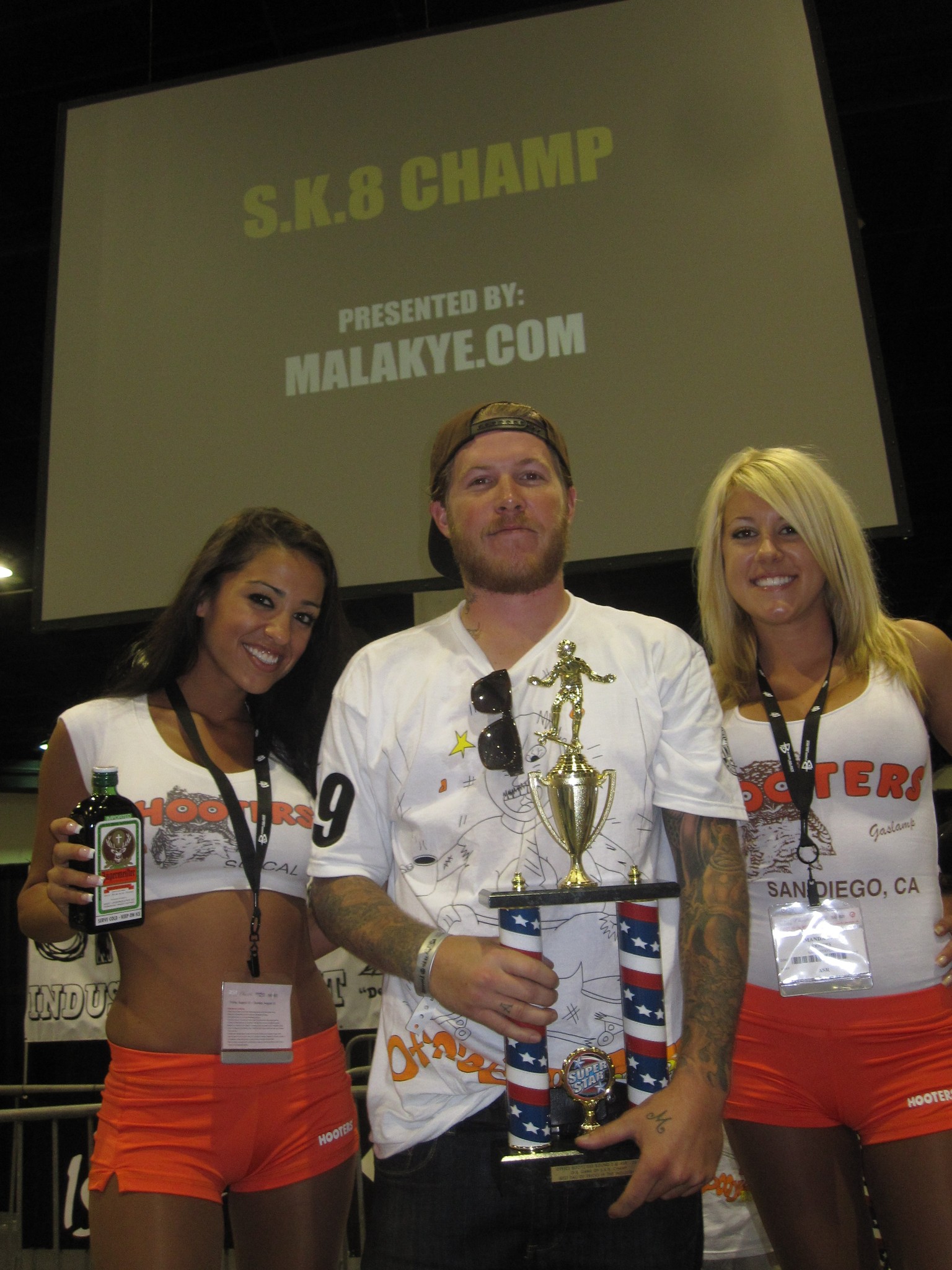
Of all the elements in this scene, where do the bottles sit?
[68,765,146,933]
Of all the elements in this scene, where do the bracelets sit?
[413,928,452,998]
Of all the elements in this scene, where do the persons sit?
[305,405,749,1270]
[17,509,359,1270]
[695,445,952,1270]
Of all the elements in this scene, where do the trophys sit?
[488,638,679,1163]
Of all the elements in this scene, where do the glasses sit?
[471,669,524,776]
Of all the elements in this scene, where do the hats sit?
[427,402,573,579]
